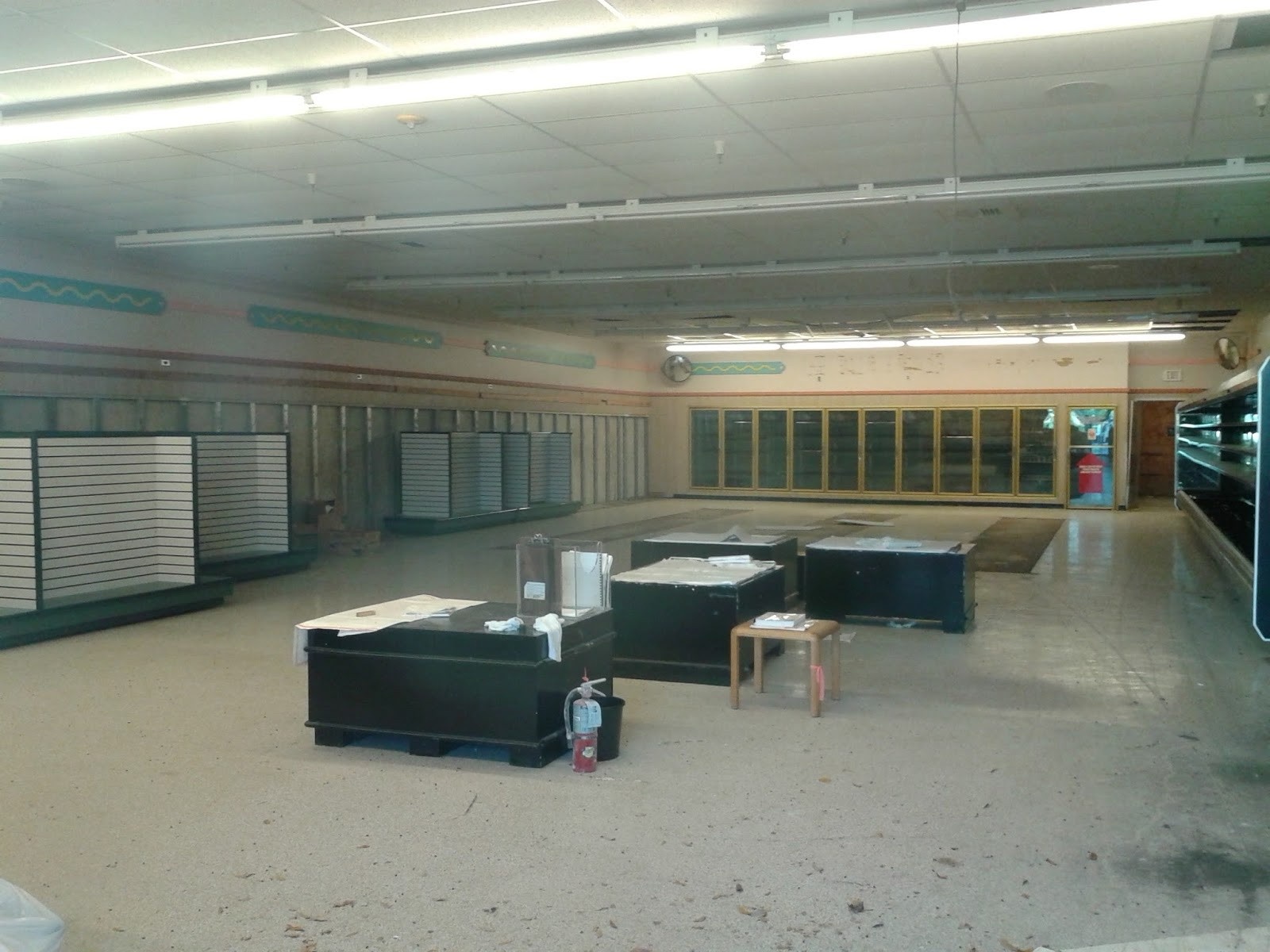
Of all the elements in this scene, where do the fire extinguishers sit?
[564,678,606,773]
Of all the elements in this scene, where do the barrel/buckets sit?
[570,697,626,761]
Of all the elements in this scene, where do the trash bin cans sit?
[569,696,626,761]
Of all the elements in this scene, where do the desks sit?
[630,529,800,609]
[301,597,612,767]
[804,532,977,636]
[605,555,784,688]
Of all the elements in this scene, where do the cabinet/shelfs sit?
[1175,382,1258,609]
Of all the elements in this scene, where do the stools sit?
[729,615,840,718]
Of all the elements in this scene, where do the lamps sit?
[0,2,1269,314]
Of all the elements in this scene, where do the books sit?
[560,552,603,608]
[753,612,806,628]
